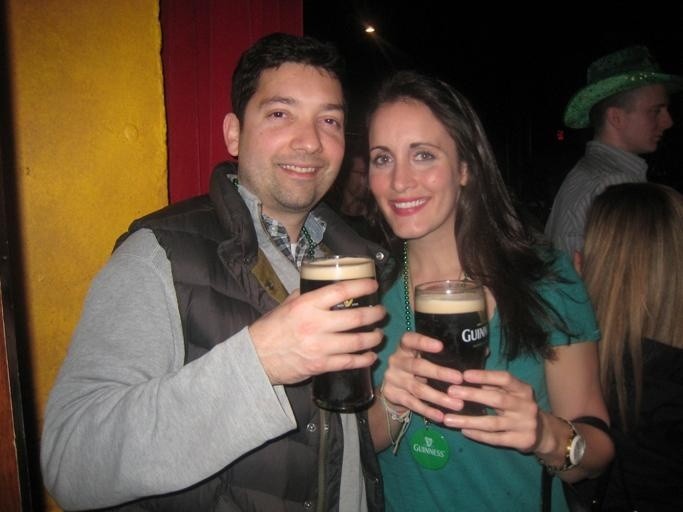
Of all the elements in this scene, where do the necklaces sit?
[402,240,468,470]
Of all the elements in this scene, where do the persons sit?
[543,42,680,264]
[573,182,683,511]
[341,147,373,219]
[37,36,398,512]
[365,68,620,512]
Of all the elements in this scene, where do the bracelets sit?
[380,388,413,456]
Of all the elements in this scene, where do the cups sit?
[299,254,379,414]
[415,279,489,429]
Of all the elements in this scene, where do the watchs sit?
[536,417,586,475]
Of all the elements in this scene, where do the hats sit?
[561,47,681,129]
